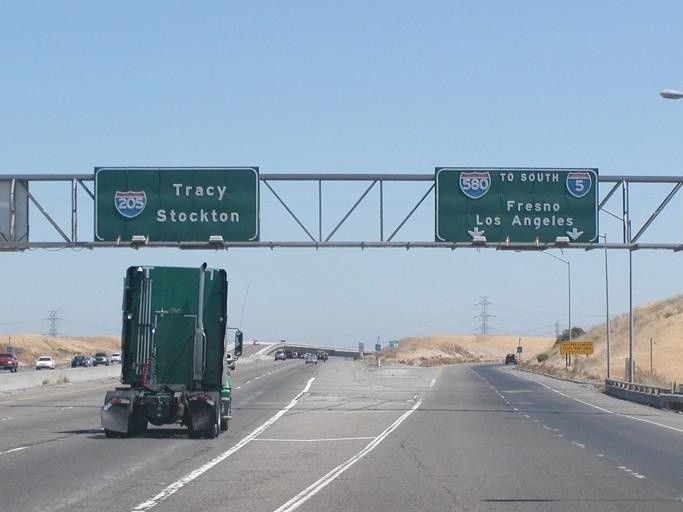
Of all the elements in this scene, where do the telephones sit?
[94,166,260,242]
[434,166,599,243]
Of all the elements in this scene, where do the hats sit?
[506,353,517,364]
[0,352,18,372]
[275,350,328,365]
[36,356,55,370]
[70,352,121,368]
